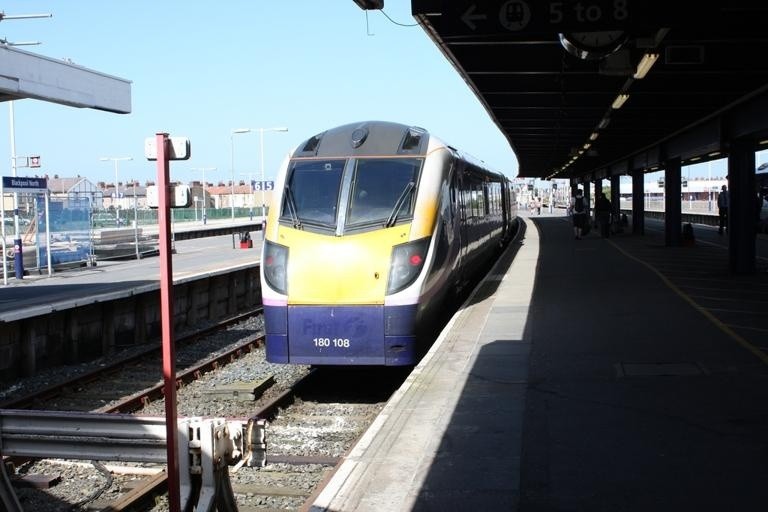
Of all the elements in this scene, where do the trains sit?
[259,118,518,372]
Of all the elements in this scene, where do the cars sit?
[0,214,31,227]
[38,214,66,225]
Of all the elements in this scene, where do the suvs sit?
[89,212,130,227]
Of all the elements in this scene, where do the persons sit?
[718,186,729,235]
[570,189,589,240]
[529,198,535,215]
[596,193,612,237]
[537,197,541,215]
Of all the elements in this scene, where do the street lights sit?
[260,126,289,220]
[229,127,254,222]
[189,167,219,224]
[239,170,263,220]
[100,156,135,227]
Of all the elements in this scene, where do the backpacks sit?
[574,195,585,212]
